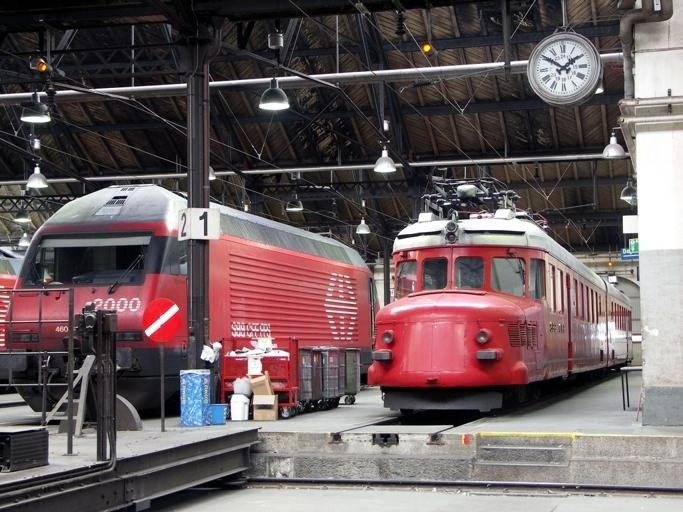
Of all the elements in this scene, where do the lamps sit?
[258,78,290,111]
[286,190,304,211]
[25,164,48,189]
[18,233,30,246]
[13,206,31,223]
[355,217,371,234]
[620,177,637,201]
[20,91,51,123]
[601,127,627,160]
[374,145,397,172]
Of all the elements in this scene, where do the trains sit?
[3,182,381,422]
[365,165,636,416]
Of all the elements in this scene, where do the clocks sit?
[526,26,605,111]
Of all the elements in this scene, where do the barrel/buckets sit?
[211,403,228,424]
[233,379,252,395]
[229,394,250,421]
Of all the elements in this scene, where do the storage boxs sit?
[247,370,279,421]
